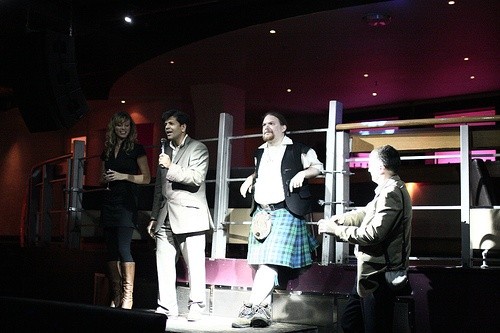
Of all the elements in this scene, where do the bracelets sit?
[150,219,157,221]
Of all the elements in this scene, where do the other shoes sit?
[157,306,178,318]
[188,306,202,321]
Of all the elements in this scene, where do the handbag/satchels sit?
[385,270,413,294]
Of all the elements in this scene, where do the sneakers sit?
[250,305,271,327]
[232,303,258,327]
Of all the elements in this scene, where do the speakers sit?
[0,297,166,333]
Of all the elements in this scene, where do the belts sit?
[259,199,286,212]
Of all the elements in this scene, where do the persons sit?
[146,109,216,324]
[316,145,413,333]
[231,112,324,329]
[98,111,151,311]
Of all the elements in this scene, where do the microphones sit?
[160,137,167,168]
